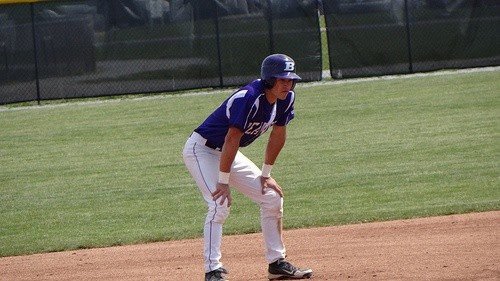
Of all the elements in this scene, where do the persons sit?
[183,53,313,281]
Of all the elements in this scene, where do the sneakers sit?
[205,268,228,281]
[268,260,312,281]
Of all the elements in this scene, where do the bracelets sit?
[261,164,273,177]
[217,170,231,184]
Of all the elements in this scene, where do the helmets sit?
[261,54,302,90]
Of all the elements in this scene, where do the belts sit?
[206,141,222,152]
